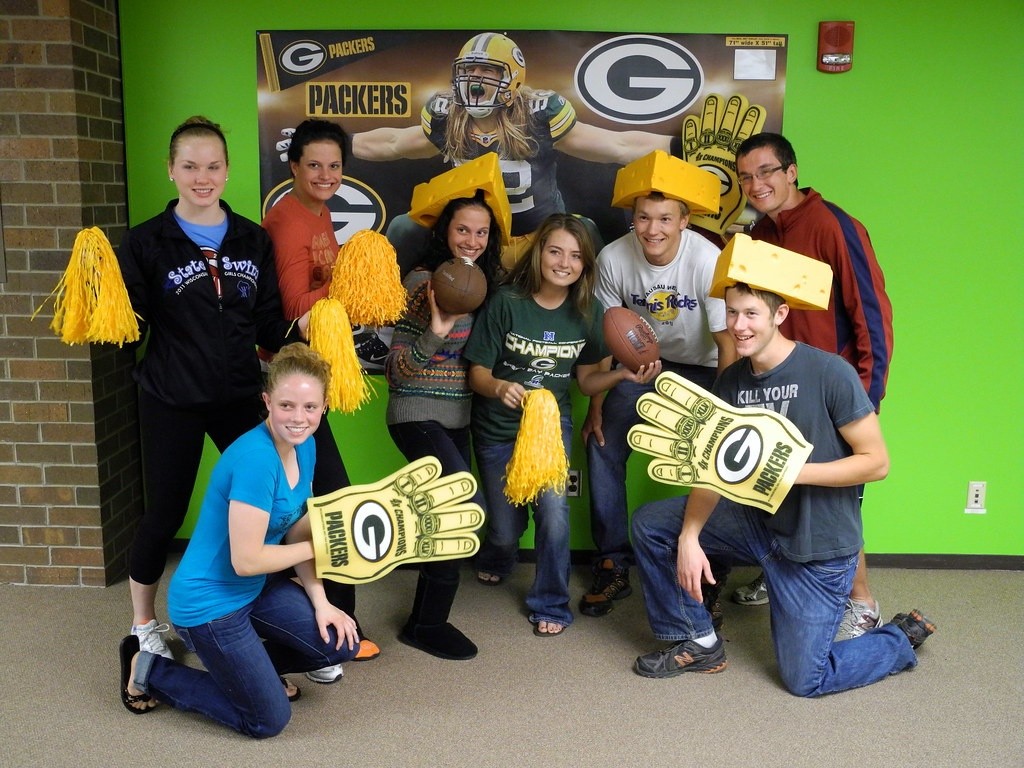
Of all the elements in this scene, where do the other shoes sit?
[350,637,381,661]
[534,621,566,636]
[477,571,503,586]
[397,614,478,660]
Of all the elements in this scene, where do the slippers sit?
[119,635,156,714]
[280,677,301,702]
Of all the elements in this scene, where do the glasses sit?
[737,164,784,184]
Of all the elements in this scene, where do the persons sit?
[629,281,937,697]
[733,132,894,642]
[578,191,742,632]
[276,32,683,281]
[384,189,509,660]
[81,116,345,683]
[257,120,381,662]
[461,214,663,638]
[119,343,360,739]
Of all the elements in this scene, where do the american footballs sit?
[431,255,488,315]
[602,306,660,374]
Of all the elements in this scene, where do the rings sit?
[512,399,516,403]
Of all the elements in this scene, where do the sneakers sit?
[305,663,344,684]
[130,619,175,660]
[700,574,727,632]
[834,597,883,641]
[580,558,632,617]
[890,609,936,650]
[634,634,727,679]
[734,571,769,605]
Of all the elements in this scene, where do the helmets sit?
[450,33,526,114]
